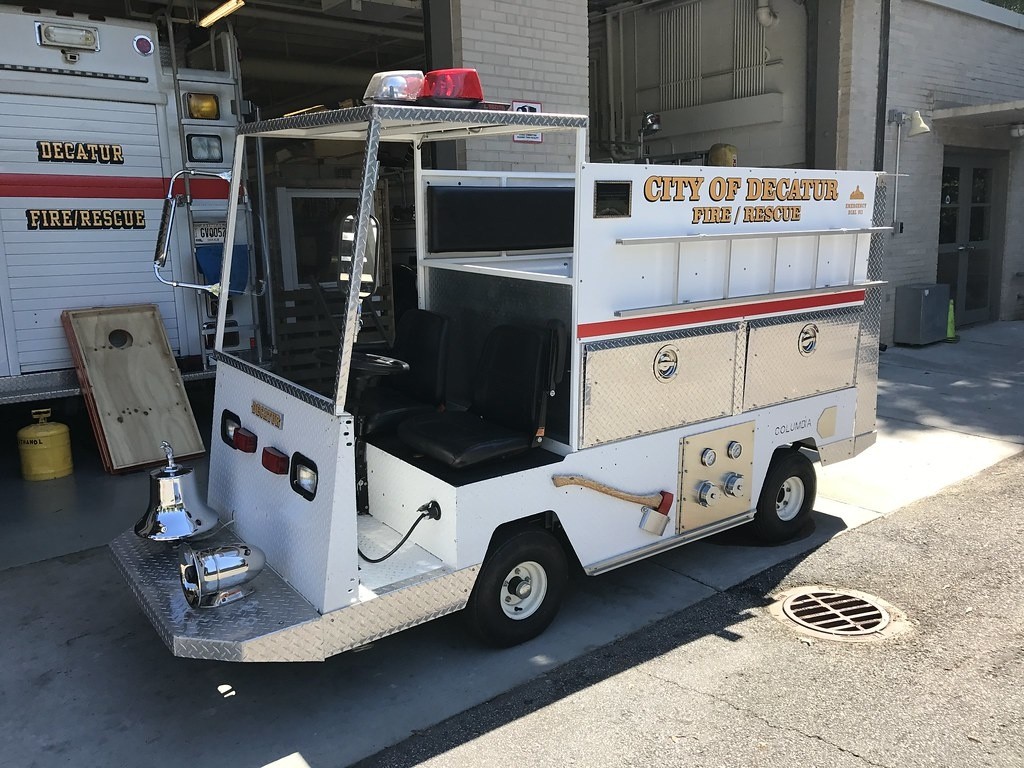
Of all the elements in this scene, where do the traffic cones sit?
[945,299,960,344]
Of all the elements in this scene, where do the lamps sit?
[901,111,930,137]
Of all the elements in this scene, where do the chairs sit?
[345,309,450,438]
[397,325,555,468]
